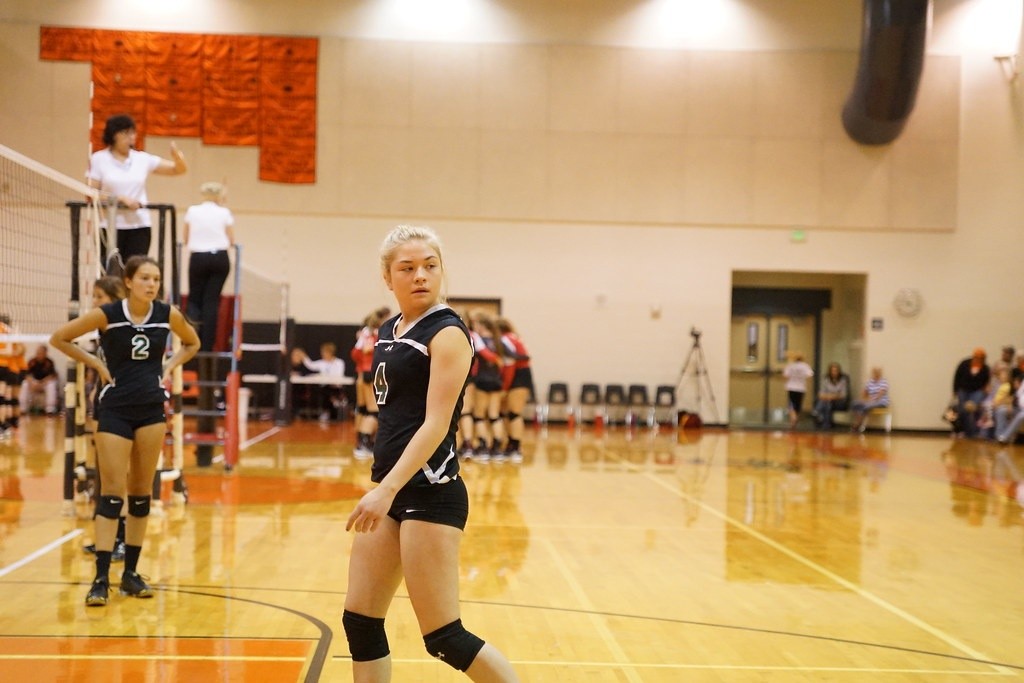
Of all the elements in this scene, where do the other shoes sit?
[20,410,30,416]
[46,407,56,415]
[84,544,95,554]
[109,539,126,562]
[353,432,375,460]
[0,419,19,444]
[455,442,525,464]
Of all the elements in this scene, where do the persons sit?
[82,276,127,562]
[341,225,519,683]
[782,350,814,428]
[259,343,346,421]
[0,316,28,436]
[85,116,187,268]
[851,367,891,432]
[182,181,235,352]
[48,255,200,605]
[951,347,1024,444]
[26,346,59,413]
[352,308,535,464]
[808,361,849,433]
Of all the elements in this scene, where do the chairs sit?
[827,374,893,436]
[538,382,677,428]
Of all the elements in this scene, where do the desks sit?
[243,376,352,421]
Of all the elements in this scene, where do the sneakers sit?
[120,571,153,597]
[85,576,112,605]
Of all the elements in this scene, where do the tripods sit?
[675,327,721,425]
